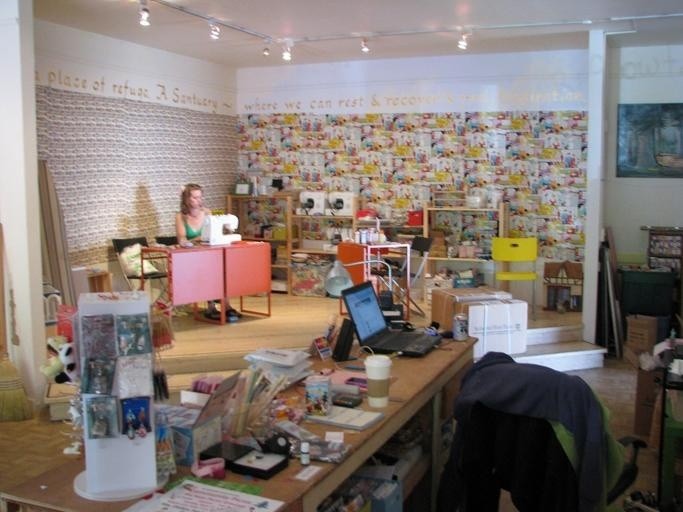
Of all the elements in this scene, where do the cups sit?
[362,354,392,406]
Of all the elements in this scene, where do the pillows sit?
[119,243,159,277]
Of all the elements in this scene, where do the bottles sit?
[299,441,310,465]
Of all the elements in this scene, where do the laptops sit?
[341,280,441,356]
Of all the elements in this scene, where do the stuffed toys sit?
[54,339,77,385]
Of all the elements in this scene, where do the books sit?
[188,373,246,398]
[307,406,383,432]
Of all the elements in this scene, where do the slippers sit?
[225,309,241,318]
[204,307,220,320]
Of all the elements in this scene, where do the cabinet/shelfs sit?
[224,193,512,323]
[612,271,682,347]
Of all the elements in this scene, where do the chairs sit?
[113,237,179,322]
[454,431,653,512]
[154,234,209,316]
[490,234,541,324]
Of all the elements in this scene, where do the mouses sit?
[441,330,453,338]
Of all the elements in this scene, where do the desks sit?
[1,329,480,511]
[653,347,682,512]
[139,242,274,324]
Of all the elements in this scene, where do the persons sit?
[38,335,68,385]
[652,105,681,168]
[174,182,243,323]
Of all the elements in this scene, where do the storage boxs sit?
[39,382,76,421]
[632,361,663,441]
[153,368,240,469]
[426,280,510,334]
[456,298,528,364]
[623,313,661,363]
[178,378,249,433]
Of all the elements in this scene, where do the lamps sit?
[138,0,270,57]
[282,25,476,64]
